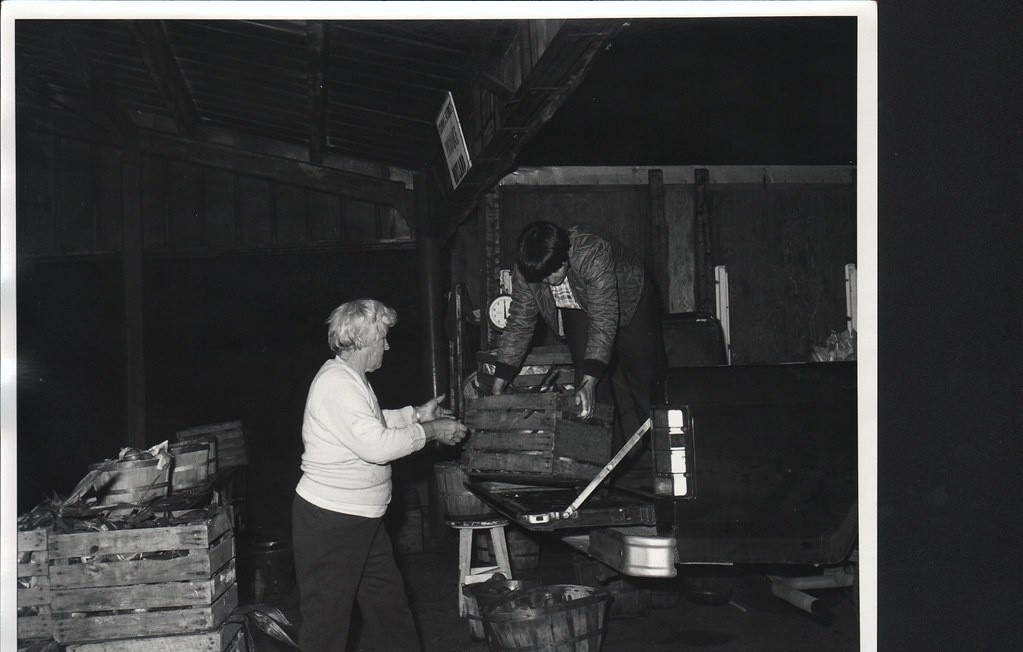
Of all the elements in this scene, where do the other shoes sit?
[613,468,654,495]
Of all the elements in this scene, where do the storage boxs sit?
[176,419,252,468]
[460,393,615,485]
[17,504,249,652]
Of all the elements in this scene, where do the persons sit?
[290,299,467,652]
[492,221,663,490]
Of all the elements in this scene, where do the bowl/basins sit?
[245,541,293,561]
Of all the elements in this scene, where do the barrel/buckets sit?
[434,460,503,522]
[471,579,542,651]
[484,584,608,651]
[479,528,540,571]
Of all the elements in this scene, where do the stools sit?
[443,519,514,618]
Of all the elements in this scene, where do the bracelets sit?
[414,405,421,424]
